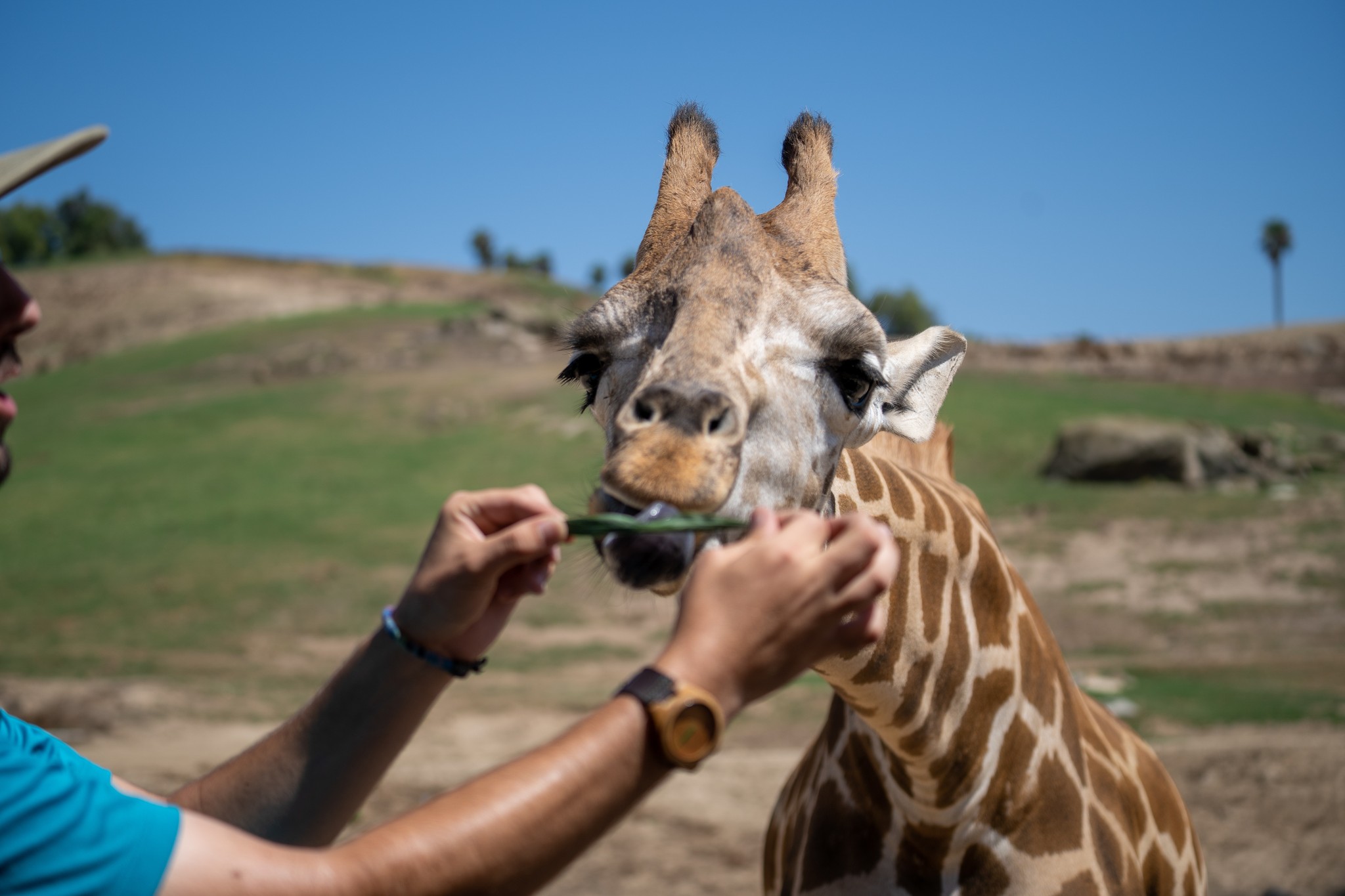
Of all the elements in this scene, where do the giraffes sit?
[553,99,1209,896]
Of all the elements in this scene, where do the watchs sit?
[614,666,724,771]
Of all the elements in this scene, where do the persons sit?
[0,126,903,896]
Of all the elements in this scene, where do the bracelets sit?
[379,608,490,679]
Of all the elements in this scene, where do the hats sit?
[0,124,109,199]
